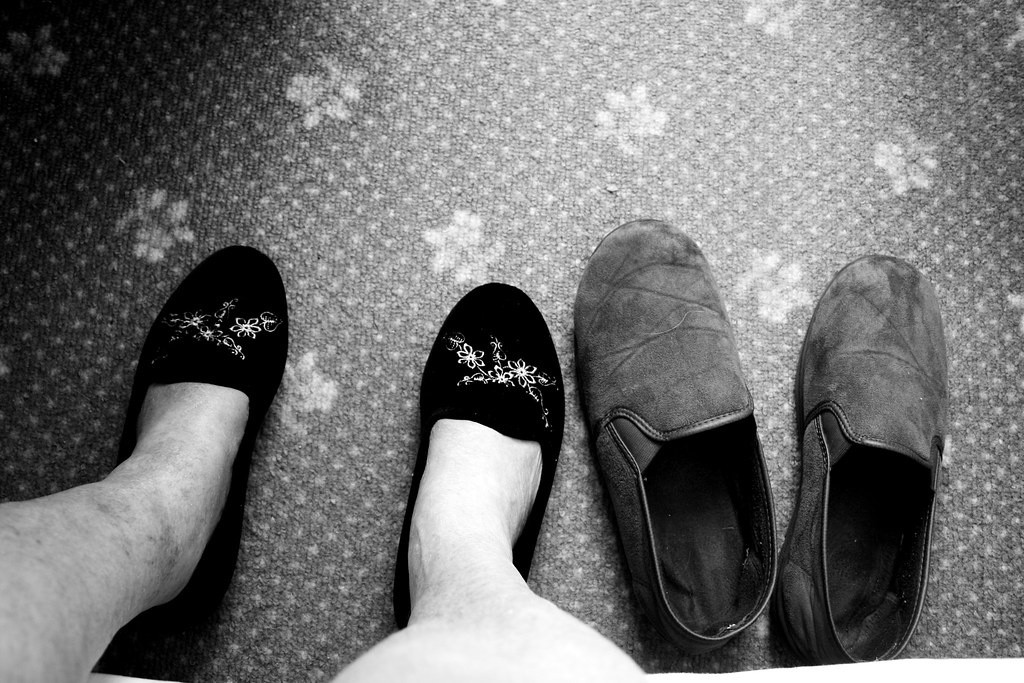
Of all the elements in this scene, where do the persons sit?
[0,246,651,683]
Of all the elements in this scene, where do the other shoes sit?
[772,254,951,668]
[576,220,773,660]
[393,282,565,633]
[121,245,288,644]
